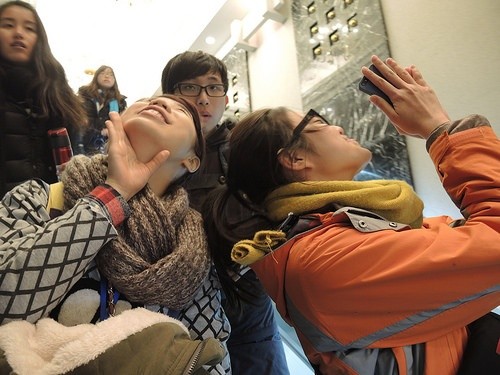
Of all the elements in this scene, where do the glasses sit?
[287,108,330,148]
[174,82,228,97]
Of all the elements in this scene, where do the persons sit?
[0,93,231,375]
[160,50,291,375]
[202,55,500,375]
[0,0,87,203]
[78,65,127,156]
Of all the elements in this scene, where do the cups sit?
[48,128,74,181]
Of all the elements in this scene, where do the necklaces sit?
[99,276,121,321]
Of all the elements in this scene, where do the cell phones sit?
[359,64,394,109]
